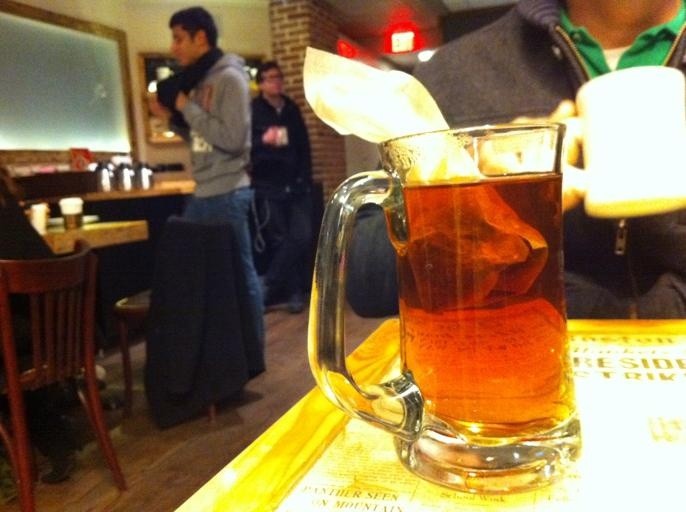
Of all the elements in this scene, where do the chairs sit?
[0,239,129,512]
[114,215,266,432]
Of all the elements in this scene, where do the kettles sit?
[94,159,154,192]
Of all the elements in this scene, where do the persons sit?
[334,0,686,320]
[252,59,315,314]
[0,165,84,484]
[148,6,266,377]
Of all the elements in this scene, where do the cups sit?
[304,122,591,496]
[29,204,47,237]
[548,62,686,227]
[156,67,174,82]
[56,195,84,231]
[276,125,290,146]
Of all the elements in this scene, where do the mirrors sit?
[136,51,268,146]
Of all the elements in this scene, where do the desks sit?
[42,219,149,390]
[174,317,686,512]
